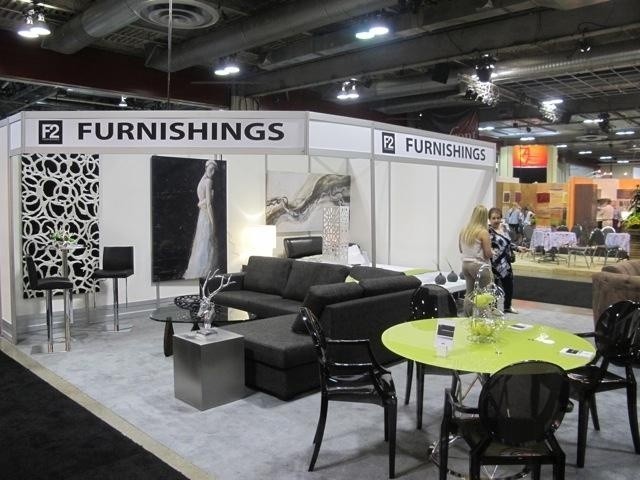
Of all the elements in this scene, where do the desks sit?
[382,317,597,480]
[421,278,467,298]
[605,232,631,260]
[38,244,85,327]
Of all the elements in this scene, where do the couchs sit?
[199,265,423,402]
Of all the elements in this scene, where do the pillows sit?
[243,256,421,335]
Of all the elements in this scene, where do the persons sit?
[181,160,220,280]
[458,204,494,317]
[487,208,533,317]
[507,201,536,249]
[611,201,622,233]
[599,200,614,229]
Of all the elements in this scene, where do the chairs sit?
[298,307,397,479]
[405,284,476,429]
[565,300,640,468]
[93,247,134,333]
[592,260,640,328]
[524,224,620,269]
[26,256,73,354]
[440,360,574,480]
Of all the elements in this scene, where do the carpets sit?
[14,303,640,480]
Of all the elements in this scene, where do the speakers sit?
[431,63,451,84]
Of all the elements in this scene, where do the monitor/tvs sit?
[513,167,547,183]
